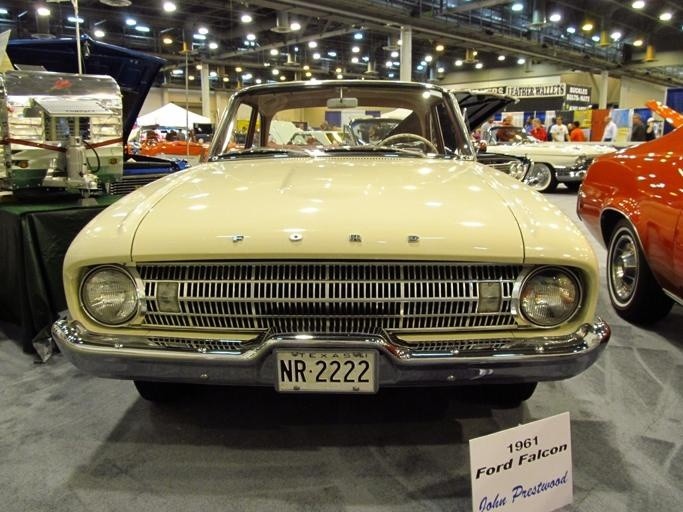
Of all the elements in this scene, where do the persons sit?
[522,113,588,144]
[480,113,498,142]
[498,115,517,141]
[629,113,646,142]
[645,117,657,141]
[599,115,616,142]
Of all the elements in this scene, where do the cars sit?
[0,34,683,193]
[50,80,613,410]
[461,126,618,191]
[575,125,682,323]
